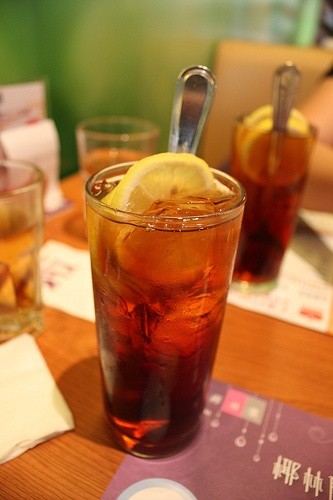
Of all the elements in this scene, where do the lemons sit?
[98,104,312,287]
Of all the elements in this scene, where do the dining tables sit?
[1,171,333,500]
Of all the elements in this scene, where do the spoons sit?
[242,61,303,278]
[106,62,218,441]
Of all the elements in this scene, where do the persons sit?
[296,62,332,210]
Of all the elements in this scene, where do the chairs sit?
[207,36,333,212]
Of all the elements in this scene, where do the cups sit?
[0,158,47,345]
[84,160,247,461]
[229,112,320,295]
[74,116,160,185]
